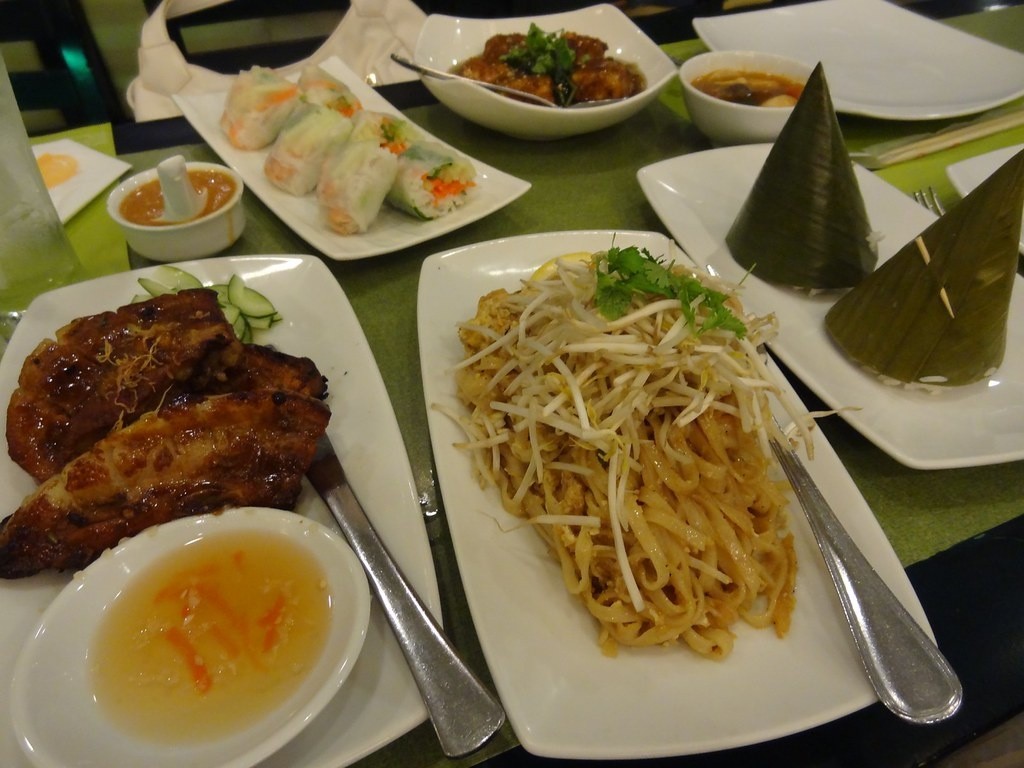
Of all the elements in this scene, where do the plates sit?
[174,55,531,260]
[4,257,445,764]
[945,145,1024,257]
[636,144,1024,469]
[691,0,1023,121]
[413,232,938,761]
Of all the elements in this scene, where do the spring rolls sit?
[219,63,476,235]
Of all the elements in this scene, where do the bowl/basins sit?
[681,50,824,147]
[107,161,245,258]
[413,2,679,140]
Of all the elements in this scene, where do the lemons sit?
[530,252,592,282]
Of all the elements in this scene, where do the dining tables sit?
[113,0,1024,768]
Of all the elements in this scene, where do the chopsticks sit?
[874,110,1024,169]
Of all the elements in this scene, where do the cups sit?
[1,51,89,320]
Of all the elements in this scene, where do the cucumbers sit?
[131,266,283,345]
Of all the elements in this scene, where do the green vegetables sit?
[594,230,748,339]
[502,23,574,76]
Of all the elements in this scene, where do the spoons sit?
[152,155,208,225]
